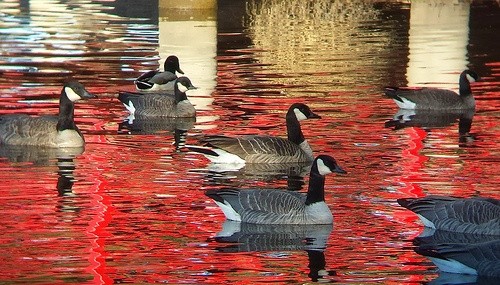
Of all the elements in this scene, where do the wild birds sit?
[184,103,323,165]
[203,154,348,225]
[397,194,500,237]
[381,70,485,110]
[0,81,98,148]
[133,55,185,92]
[115,76,198,118]
